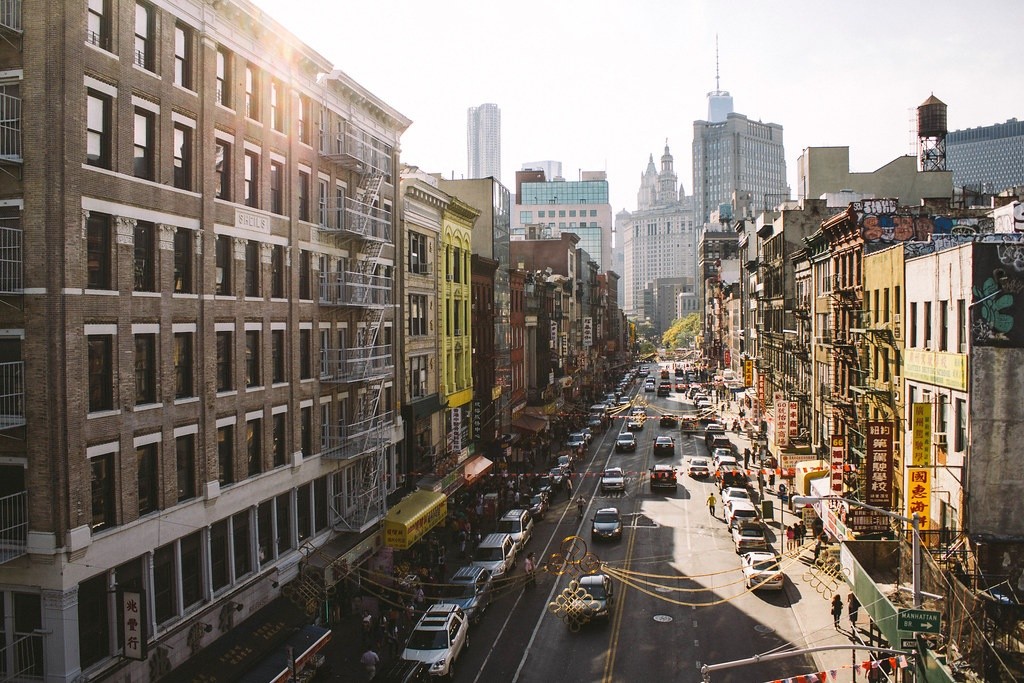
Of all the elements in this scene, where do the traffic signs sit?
[900,639,938,651]
[897,608,940,634]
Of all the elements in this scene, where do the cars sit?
[590,508,623,545]
[686,371,748,494]
[741,552,784,591]
[653,435,675,456]
[721,487,751,508]
[723,497,759,534]
[639,367,685,397]
[648,464,678,493]
[518,491,550,520]
[681,415,699,433]
[660,413,679,428]
[687,458,710,479]
[731,521,766,555]
[528,476,557,497]
[568,574,614,624]
[548,367,647,493]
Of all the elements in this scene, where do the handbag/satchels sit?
[831,609,834,615]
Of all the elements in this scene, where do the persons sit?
[433,536,448,566]
[706,493,717,516]
[552,371,625,430]
[361,584,426,657]
[744,446,775,491]
[786,521,807,551]
[456,519,483,552]
[709,383,750,435]
[813,541,828,565]
[832,594,843,630]
[479,432,589,506]
[576,495,587,511]
[360,644,379,681]
[848,589,858,628]
[525,552,537,591]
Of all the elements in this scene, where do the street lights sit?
[792,495,926,683]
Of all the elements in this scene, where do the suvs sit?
[369,508,533,683]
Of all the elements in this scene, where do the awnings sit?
[513,414,548,434]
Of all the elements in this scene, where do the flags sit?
[776,654,908,683]
